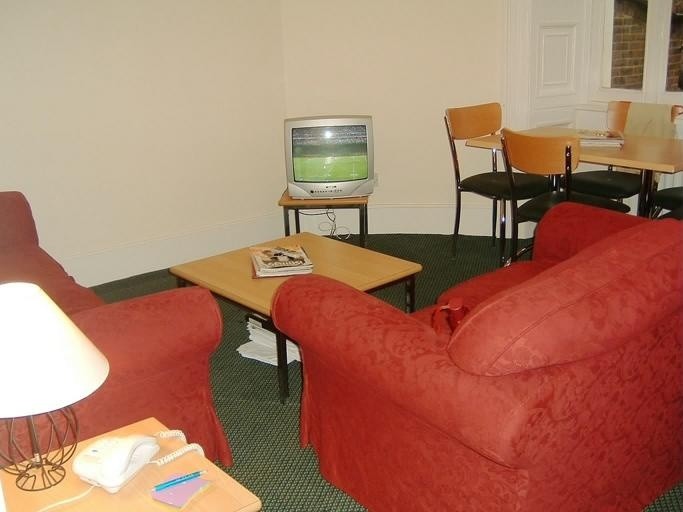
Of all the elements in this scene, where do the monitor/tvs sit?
[284,115,375,200]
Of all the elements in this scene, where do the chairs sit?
[648,185,682,219]
[443,102,553,268]
[500,127,631,262]
[560,100,682,219]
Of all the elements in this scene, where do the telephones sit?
[72,434,159,495]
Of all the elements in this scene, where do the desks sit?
[465,126,682,269]
[278,186,368,249]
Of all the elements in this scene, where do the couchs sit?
[1,190,235,468]
[268,202,683,509]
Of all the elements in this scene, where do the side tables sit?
[0,415,265,512]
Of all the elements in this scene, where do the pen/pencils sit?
[151,471,208,492]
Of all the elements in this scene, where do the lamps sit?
[1,280,111,488]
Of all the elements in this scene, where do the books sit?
[577,129,625,148]
[237,311,301,366]
[249,244,313,281]
